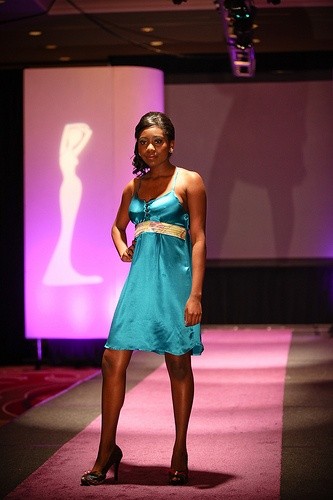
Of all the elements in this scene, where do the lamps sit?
[233,53,250,73]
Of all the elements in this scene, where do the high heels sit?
[80,444,123,485]
[169,450,189,485]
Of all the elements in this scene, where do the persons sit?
[79,111,205,487]
